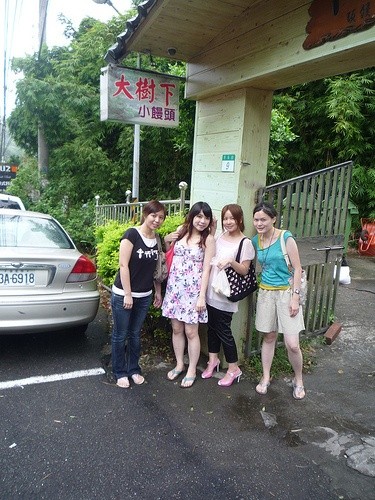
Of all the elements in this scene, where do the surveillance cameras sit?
[168,47,176,56]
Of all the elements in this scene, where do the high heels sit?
[217,366,242,386]
[201,358,220,378]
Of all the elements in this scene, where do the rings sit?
[174,237,175,239]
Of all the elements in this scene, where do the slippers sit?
[255,379,270,394]
[291,377,305,399]
[166,365,185,380]
[180,372,196,387]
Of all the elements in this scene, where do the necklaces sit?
[262,227,276,269]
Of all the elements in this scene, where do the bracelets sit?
[231,258,234,267]
[293,289,300,294]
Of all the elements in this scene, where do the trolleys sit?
[357,217,375,260]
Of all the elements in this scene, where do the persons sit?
[162,201,216,389]
[202,204,255,387]
[111,199,166,389]
[251,201,307,400]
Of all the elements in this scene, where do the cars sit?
[0,193,26,211]
[0,208,101,336]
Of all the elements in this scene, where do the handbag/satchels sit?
[223,236,257,302]
[209,255,230,297]
[154,233,168,282]
[281,229,307,305]
[165,243,173,274]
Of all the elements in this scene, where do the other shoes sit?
[116,376,129,388]
[132,373,144,384]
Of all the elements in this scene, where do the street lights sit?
[92,0,141,224]
